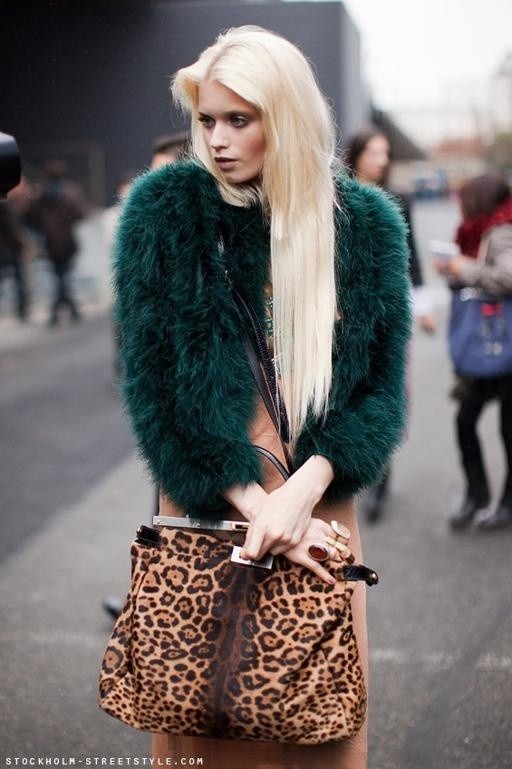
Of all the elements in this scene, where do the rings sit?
[330,519,352,541]
[307,543,330,563]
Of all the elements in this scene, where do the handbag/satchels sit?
[93,523,380,750]
[446,280,512,387]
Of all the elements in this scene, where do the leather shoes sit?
[361,473,511,530]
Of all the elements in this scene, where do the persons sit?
[347,126,436,524]
[0,172,33,324]
[21,154,92,328]
[112,21,414,769]
[432,174,512,533]
